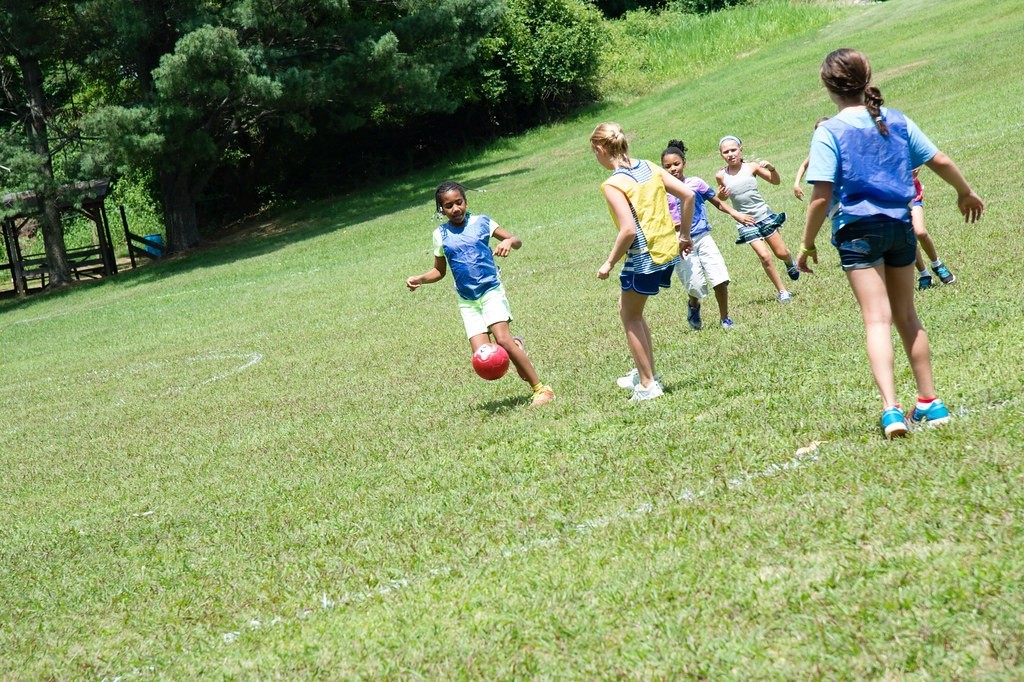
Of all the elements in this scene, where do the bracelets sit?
[769,166,775,172]
[801,244,816,254]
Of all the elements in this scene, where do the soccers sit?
[472,343,510,381]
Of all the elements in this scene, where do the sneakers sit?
[779,289,791,301]
[918,276,932,290]
[931,263,955,283]
[627,382,665,402]
[617,369,663,389]
[687,299,701,330]
[719,319,734,328]
[513,336,525,353]
[906,398,949,429]
[785,260,799,281]
[528,384,554,408]
[881,405,908,439]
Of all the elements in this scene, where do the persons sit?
[661,138,755,331]
[715,136,799,303]
[794,49,986,439]
[405,180,556,408]
[590,120,695,403]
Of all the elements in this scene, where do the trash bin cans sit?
[144,234,162,257]
